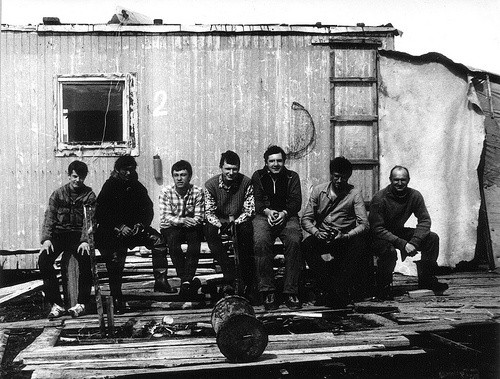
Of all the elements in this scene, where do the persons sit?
[250,145,302,310]
[366,166,449,303]
[300,155,371,302]
[159,159,208,290]
[37,160,98,319]
[202,149,255,296]
[93,153,179,316]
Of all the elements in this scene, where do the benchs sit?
[55,237,360,309]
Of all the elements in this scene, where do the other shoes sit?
[154,276,177,293]
[372,282,387,295]
[264,293,278,310]
[180,278,199,297]
[111,290,130,308]
[282,293,300,310]
[426,278,449,291]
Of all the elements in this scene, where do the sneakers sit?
[68,303,84,317]
[48,302,67,319]
